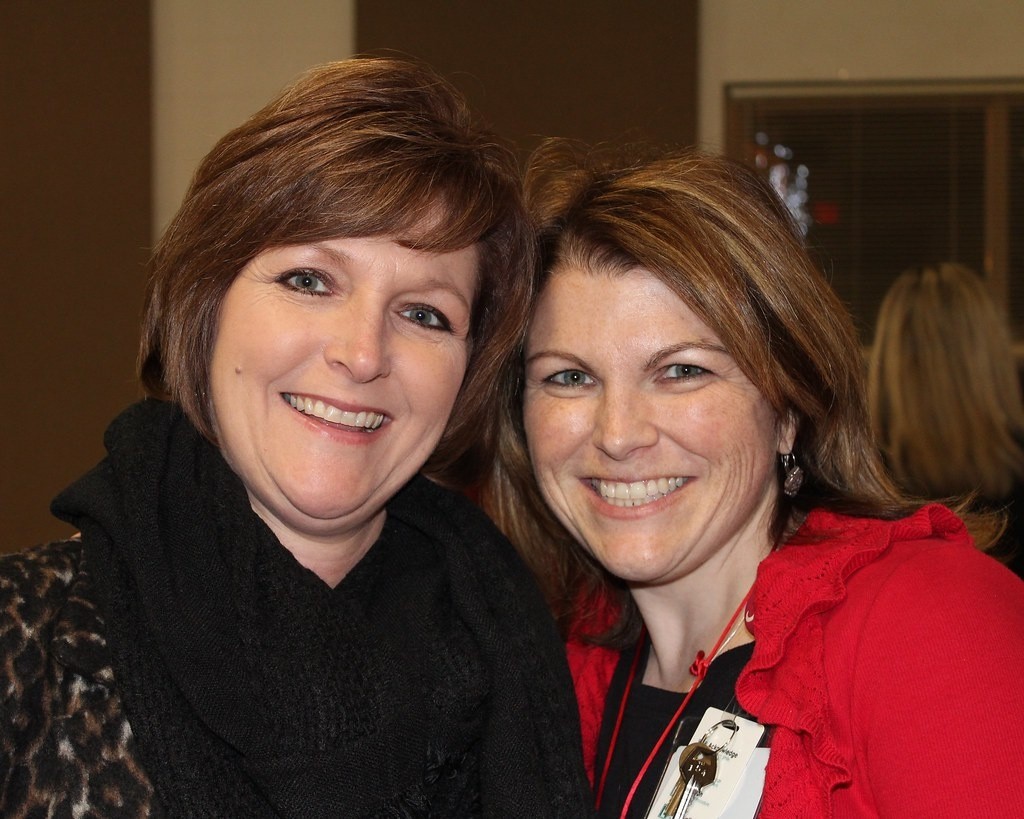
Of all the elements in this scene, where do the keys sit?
[661,717,736,819]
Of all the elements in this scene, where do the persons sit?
[514,134,1023,819]
[0,56,609,818]
[856,256,1023,585]
